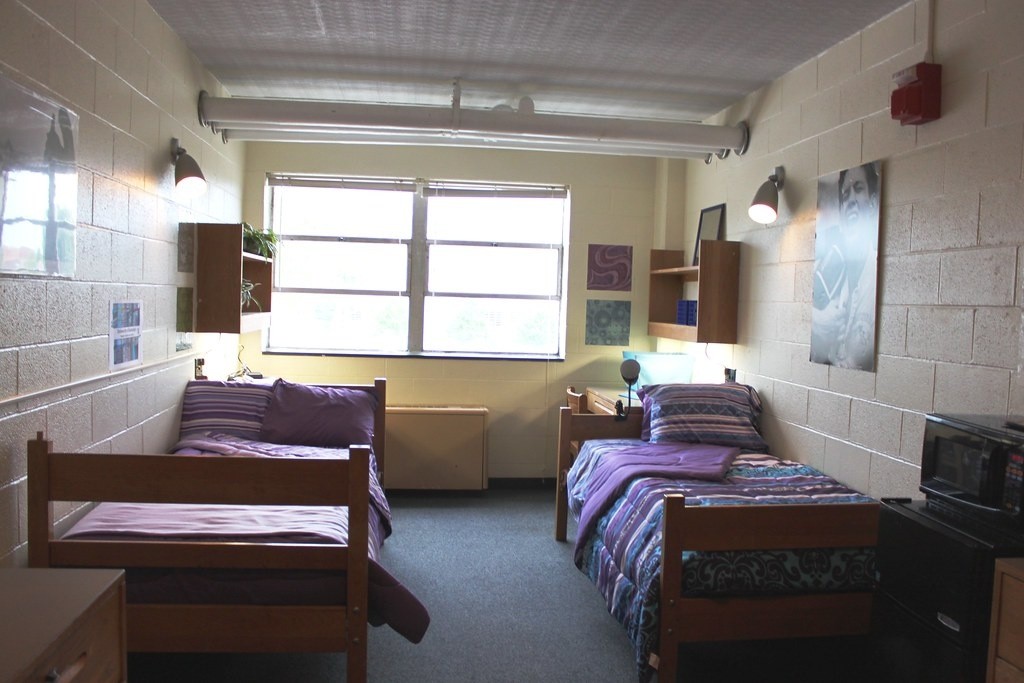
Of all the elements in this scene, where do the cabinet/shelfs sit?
[0,567,127,683]
[195,222,273,334]
[646,239,741,344]
[985,557,1024,683]
[875,498,1024,682]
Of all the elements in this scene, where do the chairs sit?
[566,384,588,470]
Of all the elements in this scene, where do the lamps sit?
[170,137,209,203]
[614,357,641,421]
[747,165,785,223]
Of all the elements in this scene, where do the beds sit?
[27,377,431,683]
[555,407,880,682]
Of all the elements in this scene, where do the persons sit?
[809,163,879,373]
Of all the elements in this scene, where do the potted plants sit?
[237,221,285,265]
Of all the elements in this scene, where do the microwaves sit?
[919,412,1024,539]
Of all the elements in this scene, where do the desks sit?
[585,384,644,416]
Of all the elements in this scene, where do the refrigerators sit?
[862,490,1024,683]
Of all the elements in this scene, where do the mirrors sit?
[692,202,725,266]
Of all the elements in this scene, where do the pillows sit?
[179,379,273,442]
[643,383,770,449]
[262,376,377,447]
[635,388,653,441]
